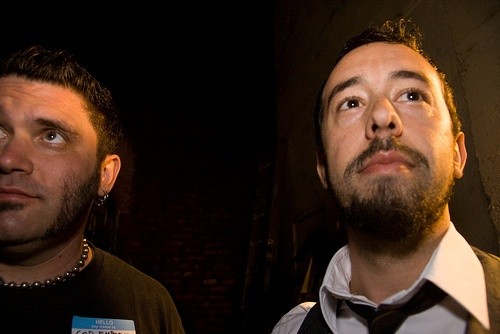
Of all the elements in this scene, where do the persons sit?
[266,16,500,334]
[0,44,186,334]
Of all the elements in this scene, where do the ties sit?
[344,279,449,334]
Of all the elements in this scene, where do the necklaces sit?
[0,234,89,291]
